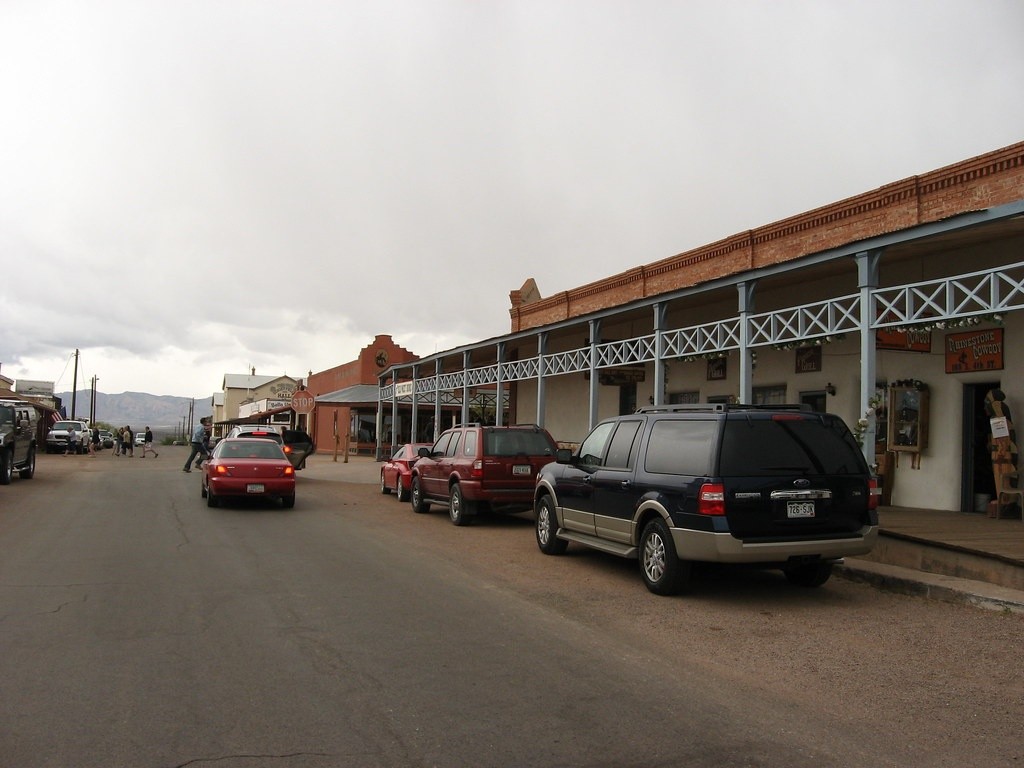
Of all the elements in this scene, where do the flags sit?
[51,406,67,421]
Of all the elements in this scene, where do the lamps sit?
[825,383,836,395]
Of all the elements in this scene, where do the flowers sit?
[769,332,847,351]
[751,350,759,370]
[679,350,731,362]
[896,312,1008,332]
[853,391,882,446]
[664,361,671,396]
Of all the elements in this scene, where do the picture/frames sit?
[706,359,727,380]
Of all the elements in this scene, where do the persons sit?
[280,425,306,469]
[88,425,101,457]
[139,425,158,458]
[61,426,78,458]
[182,417,216,473]
[114,424,135,457]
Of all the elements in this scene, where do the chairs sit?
[996,467,1024,522]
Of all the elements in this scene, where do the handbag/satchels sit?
[66,437,71,443]
[144,442,151,452]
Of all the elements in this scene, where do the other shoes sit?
[183,468,191,473]
[196,465,203,470]
[141,456,145,458]
[155,454,158,458]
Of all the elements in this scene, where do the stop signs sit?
[291,390,316,415]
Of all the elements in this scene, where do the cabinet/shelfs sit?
[887,383,930,459]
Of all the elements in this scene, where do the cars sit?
[87,427,113,451]
[200,437,296,508]
[134,432,147,447]
[380,443,434,503]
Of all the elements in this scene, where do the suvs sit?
[45,421,90,455]
[215,422,316,471]
[533,403,880,597]
[410,422,561,526]
[0,399,37,486]
